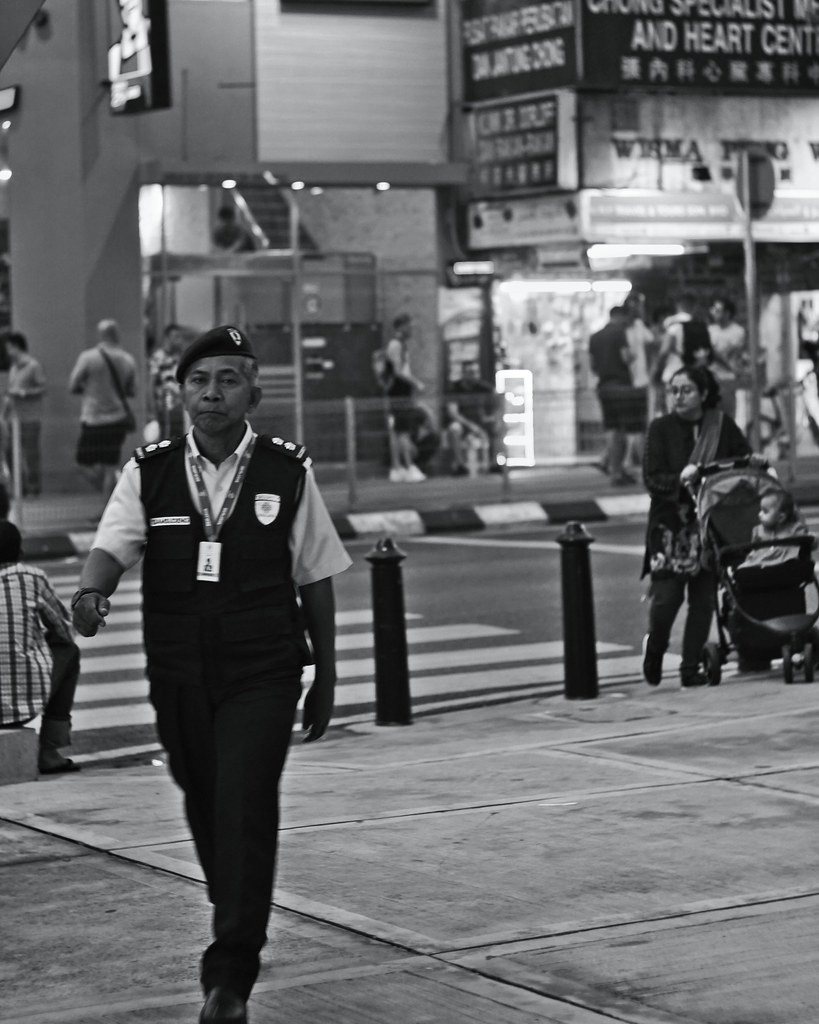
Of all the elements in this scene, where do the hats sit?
[176,324,259,386]
[391,313,411,332]
[372,352,392,379]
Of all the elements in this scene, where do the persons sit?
[737,490,809,568]
[383,314,428,483]
[443,360,501,477]
[0,521,82,775]
[71,326,353,1024]
[2,333,47,499]
[588,290,746,487]
[67,318,138,522]
[643,366,768,687]
[148,324,187,441]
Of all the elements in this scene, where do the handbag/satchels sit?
[124,410,140,431]
[648,521,705,580]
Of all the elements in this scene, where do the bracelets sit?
[72,587,107,611]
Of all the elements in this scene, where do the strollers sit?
[683,456,819,686]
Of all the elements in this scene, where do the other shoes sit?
[403,465,428,483]
[679,666,709,687]
[38,756,78,775]
[642,636,664,686]
[196,986,248,1024]
[388,467,407,483]
